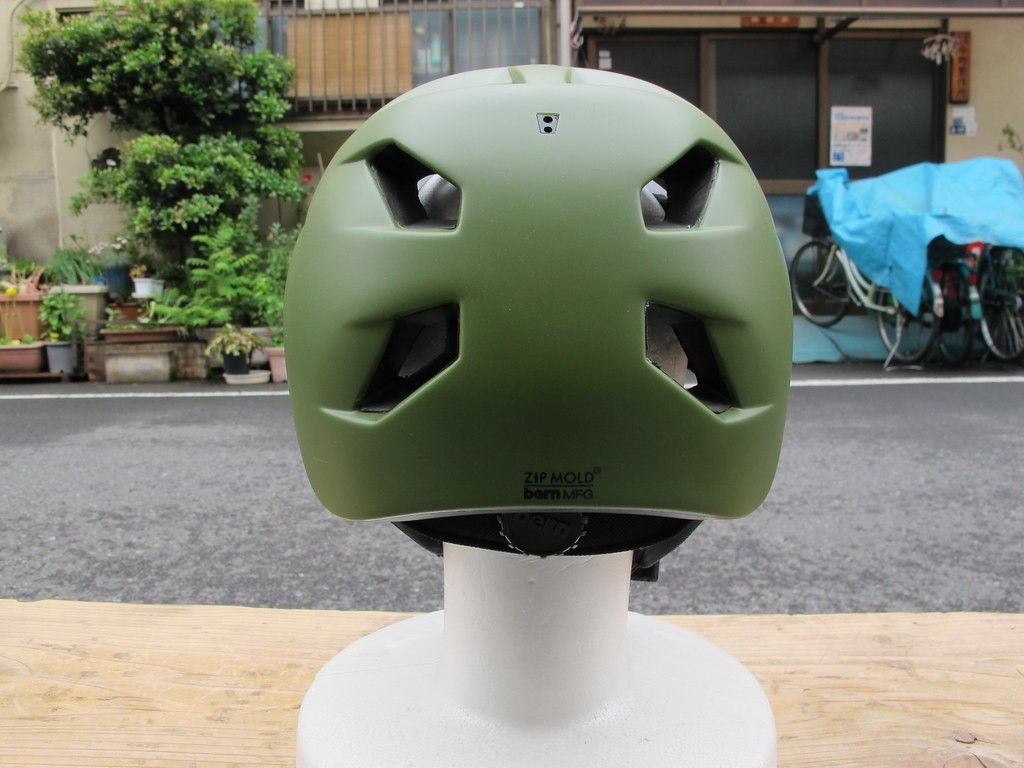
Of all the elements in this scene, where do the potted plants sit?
[0,225,302,384]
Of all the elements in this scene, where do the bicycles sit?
[790,194,1024,373]
